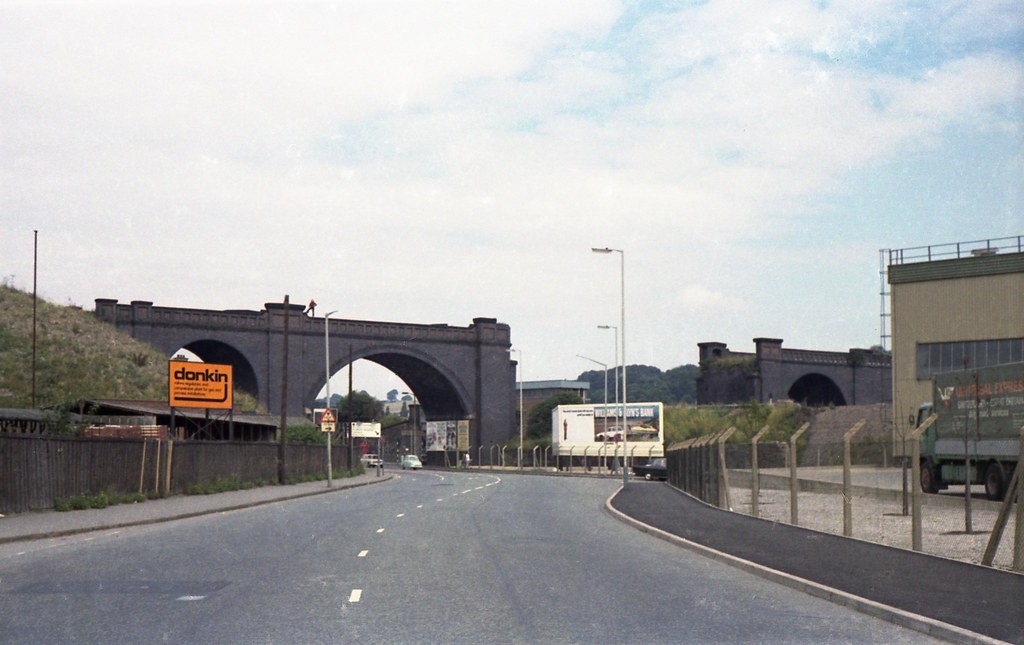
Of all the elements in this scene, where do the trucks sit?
[909,360,1024,501]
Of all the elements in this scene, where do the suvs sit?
[597,426,624,442]
[360,454,383,468]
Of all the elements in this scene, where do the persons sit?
[462,452,470,468]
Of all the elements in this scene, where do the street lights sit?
[323,310,337,486]
[507,349,523,471]
[591,247,627,489]
[575,355,608,476]
[596,325,618,475]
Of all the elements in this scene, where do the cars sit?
[633,458,667,481]
[631,424,657,434]
[402,455,422,470]
